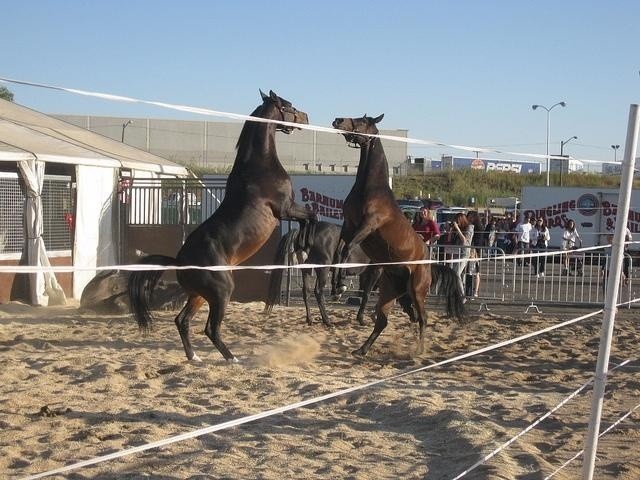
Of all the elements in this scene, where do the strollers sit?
[564,237,586,278]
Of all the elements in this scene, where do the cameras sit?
[449,222,453,227]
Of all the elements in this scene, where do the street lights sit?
[560,135,578,187]
[610,143,621,162]
[532,97,567,188]
[121,119,133,143]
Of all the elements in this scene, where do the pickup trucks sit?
[161,192,200,206]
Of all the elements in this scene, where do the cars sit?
[393,195,517,257]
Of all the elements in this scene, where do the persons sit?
[404,207,551,297]
[561,220,583,273]
[603,217,633,290]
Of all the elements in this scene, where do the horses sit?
[265,220,389,329]
[331,113,468,361]
[127,89,320,362]
